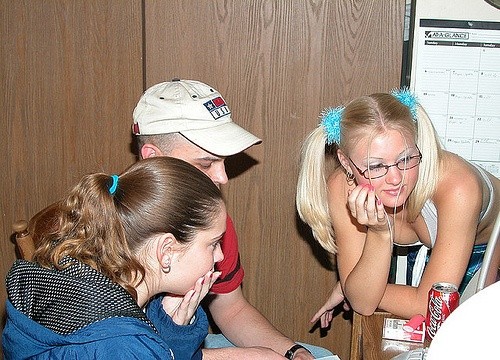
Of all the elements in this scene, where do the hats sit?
[131,77,263,158]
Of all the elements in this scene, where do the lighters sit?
[403,314,426,333]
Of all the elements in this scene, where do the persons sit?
[0,156,228,360]
[296,91,500,329]
[131,79,341,360]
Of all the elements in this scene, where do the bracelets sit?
[285,344,311,360]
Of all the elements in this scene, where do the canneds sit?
[424,281,460,342]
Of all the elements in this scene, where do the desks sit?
[350,308,429,360]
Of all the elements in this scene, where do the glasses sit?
[347,144,423,180]
